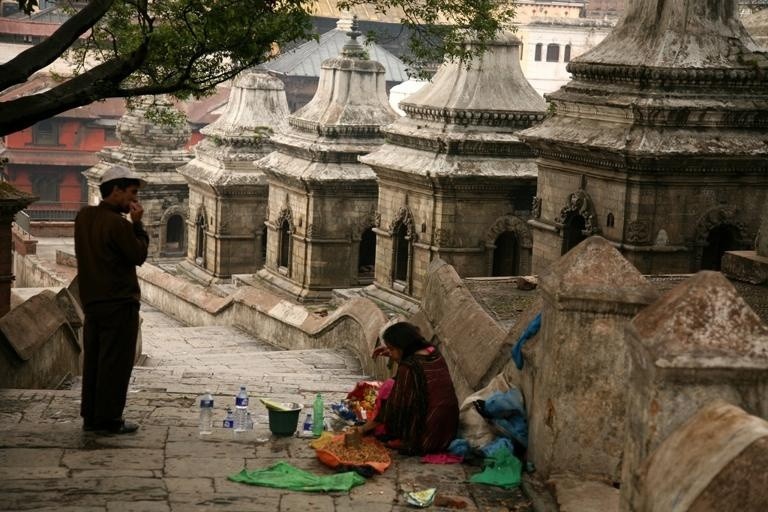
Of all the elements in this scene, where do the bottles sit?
[304,390,325,435]
[223,386,254,432]
[199,389,215,435]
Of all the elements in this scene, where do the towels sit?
[472,445,524,492]
[226,461,366,495]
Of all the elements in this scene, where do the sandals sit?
[349,424,381,435]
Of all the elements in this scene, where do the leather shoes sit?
[98,421,140,436]
[81,419,99,430]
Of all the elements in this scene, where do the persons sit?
[74,165,149,434]
[348,320,460,455]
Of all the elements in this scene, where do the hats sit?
[97,164,147,189]
